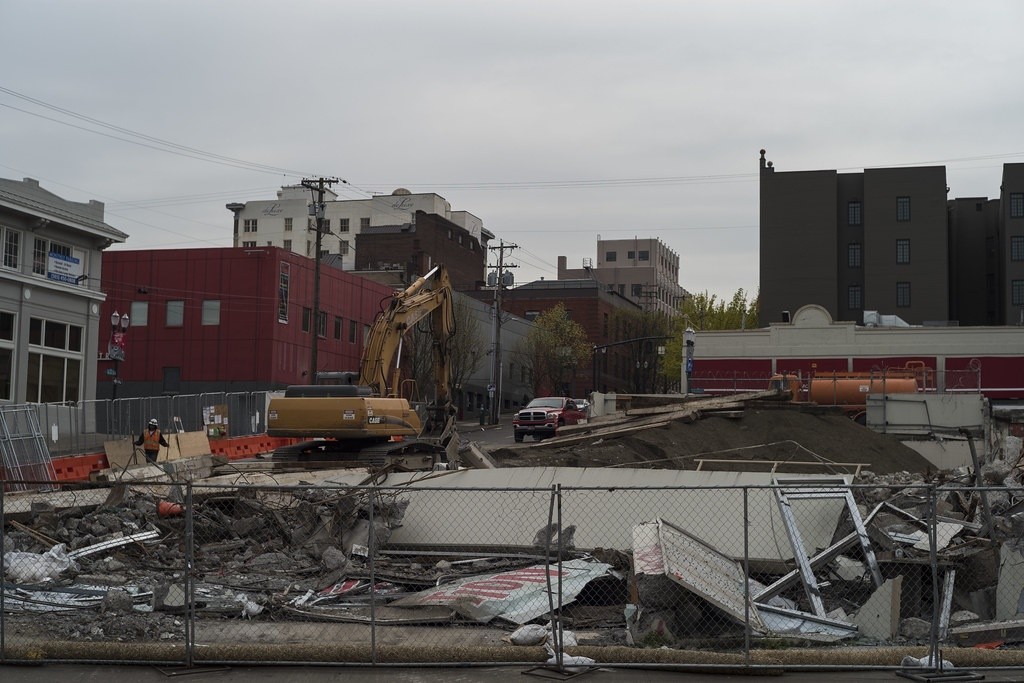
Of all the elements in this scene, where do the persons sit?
[479,404,485,427]
[134,419,171,463]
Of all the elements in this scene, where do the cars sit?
[574,398,589,416]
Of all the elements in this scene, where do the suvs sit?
[514,395,583,441]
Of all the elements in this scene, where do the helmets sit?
[149,418,158,426]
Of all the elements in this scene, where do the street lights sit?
[107,310,135,402]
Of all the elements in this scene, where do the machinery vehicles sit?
[253,264,472,473]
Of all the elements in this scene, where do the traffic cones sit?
[156,497,186,519]
